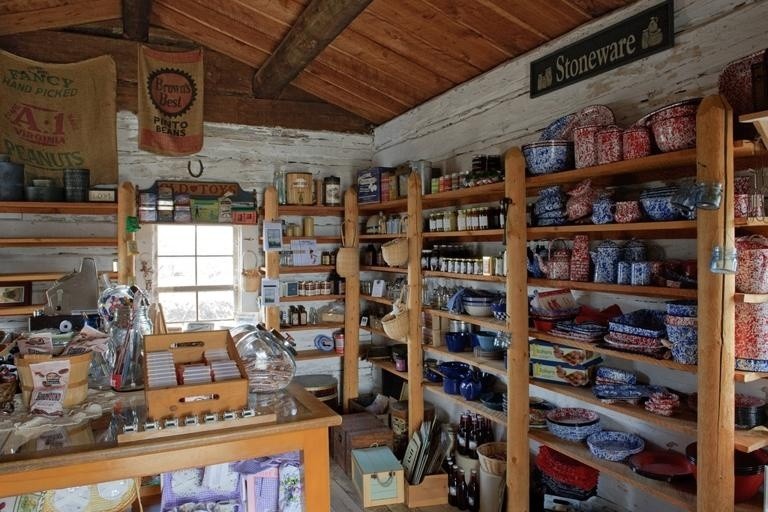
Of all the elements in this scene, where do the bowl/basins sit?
[461,295,498,318]
[520,141,572,174]
[440,331,504,411]
[691,463,764,503]
[640,98,704,151]
[25,179,56,201]
[532,179,682,226]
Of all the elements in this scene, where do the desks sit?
[2,331,343,512]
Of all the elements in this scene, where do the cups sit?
[571,125,649,169]
[632,262,651,288]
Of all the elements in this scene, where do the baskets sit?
[382,215,409,267]
[478,442,507,475]
[336,220,361,278]
[243,251,260,291]
[381,287,409,340]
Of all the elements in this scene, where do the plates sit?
[530,301,700,367]
[733,393,766,430]
[535,106,617,142]
[529,336,697,424]
[530,398,697,493]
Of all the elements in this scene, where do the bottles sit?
[230,322,297,394]
[417,155,509,277]
[108,306,154,392]
[273,171,285,204]
[363,210,402,266]
[324,176,341,207]
[280,250,346,326]
[281,216,314,238]
[442,409,493,511]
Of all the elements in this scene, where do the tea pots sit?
[524,235,649,282]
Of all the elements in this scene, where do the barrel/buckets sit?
[241,248,262,293]
[388,397,438,458]
[285,173,317,206]
[0,157,26,202]
[478,466,507,510]
[297,373,340,466]
[12,350,95,411]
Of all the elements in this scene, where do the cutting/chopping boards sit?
[286,173,313,207]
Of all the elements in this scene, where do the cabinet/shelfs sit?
[263,98,768,512]
[1,184,141,352]
